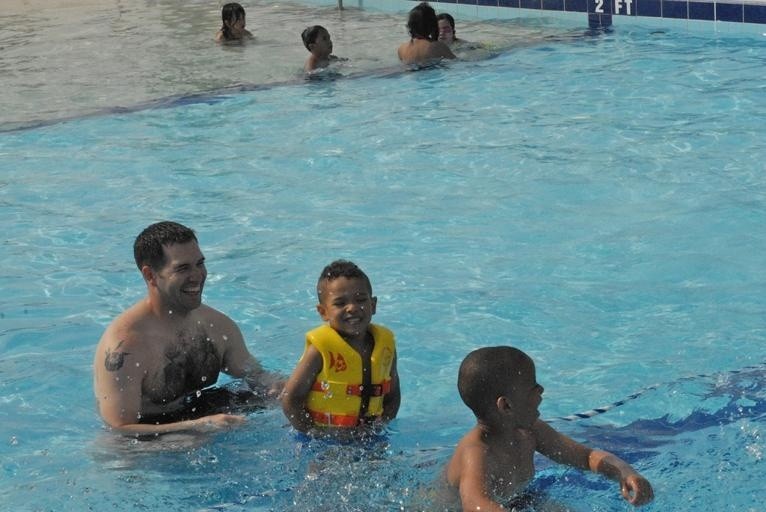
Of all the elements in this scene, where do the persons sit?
[213,2,257,47]
[89,219,288,460]
[408,346,654,511]
[397,2,457,76]
[435,13,482,54]
[276,258,402,481]
[300,24,349,80]
